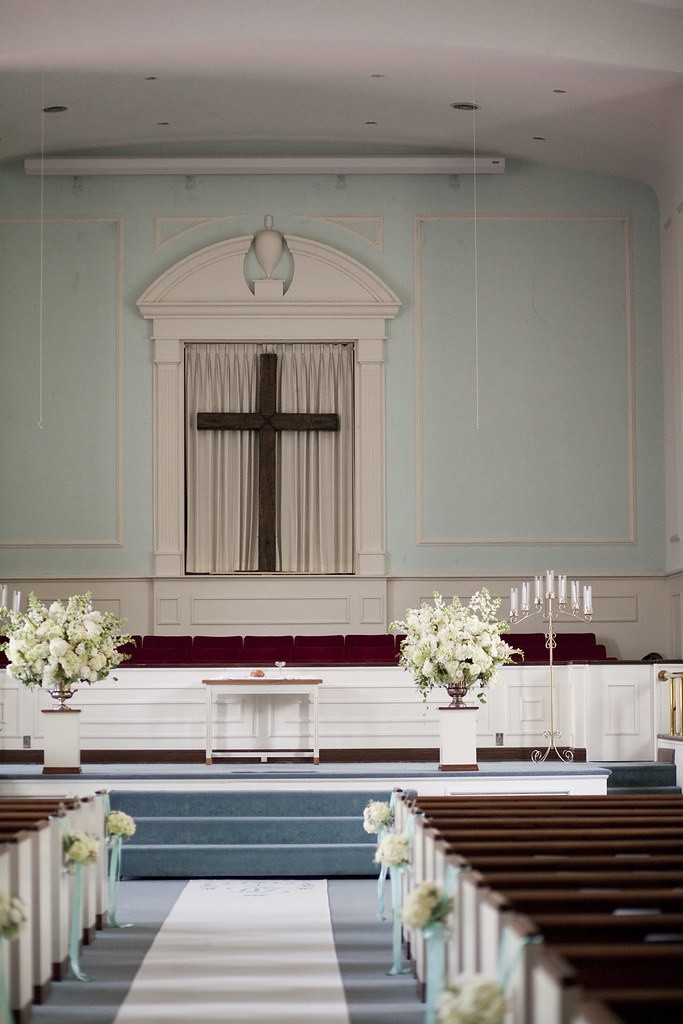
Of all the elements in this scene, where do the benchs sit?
[0,789,111,1024]
[389,787,683,1024]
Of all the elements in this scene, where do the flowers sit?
[0,891,29,943]
[363,798,395,834]
[107,811,137,847]
[62,834,100,875]
[384,587,525,726]
[438,970,508,1024]
[401,879,454,930]
[0,591,138,692]
[373,831,411,868]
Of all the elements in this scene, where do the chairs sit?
[0,632,619,671]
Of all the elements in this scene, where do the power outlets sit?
[496,733,503,746]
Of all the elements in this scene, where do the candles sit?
[571,580,581,604]
[535,575,544,598]
[510,587,518,611]
[522,581,530,605]
[559,575,567,598]
[583,586,593,609]
[546,570,555,592]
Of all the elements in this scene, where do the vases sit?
[46,682,78,710]
[447,680,467,708]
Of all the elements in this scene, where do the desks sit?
[202,679,324,766]
